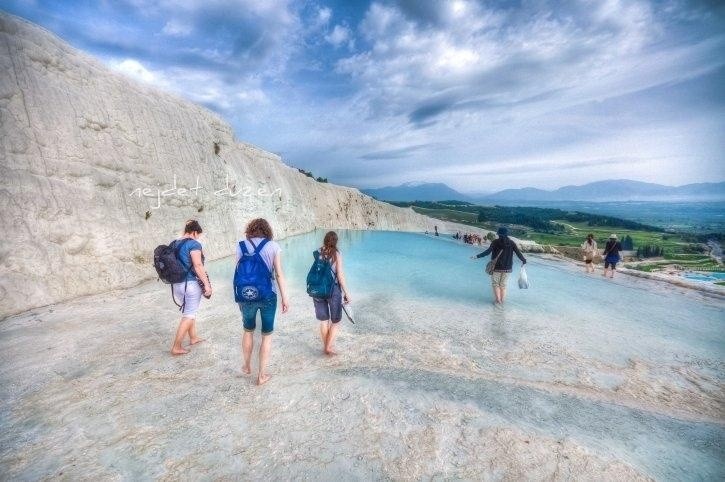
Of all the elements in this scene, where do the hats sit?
[609,234,618,238]
[497,228,507,236]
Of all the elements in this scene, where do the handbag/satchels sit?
[485,260,496,275]
[601,254,607,260]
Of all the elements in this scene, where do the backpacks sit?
[233,239,272,303]
[154,238,197,284]
[306,250,337,300]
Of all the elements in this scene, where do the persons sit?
[581,234,599,273]
[306,231,352,355]
[425,225,439,236]
[154,220,212,354]
[601,234,622,278]
[454,232,481,245]
[470,226,527,305]
[233,218,289,387]
